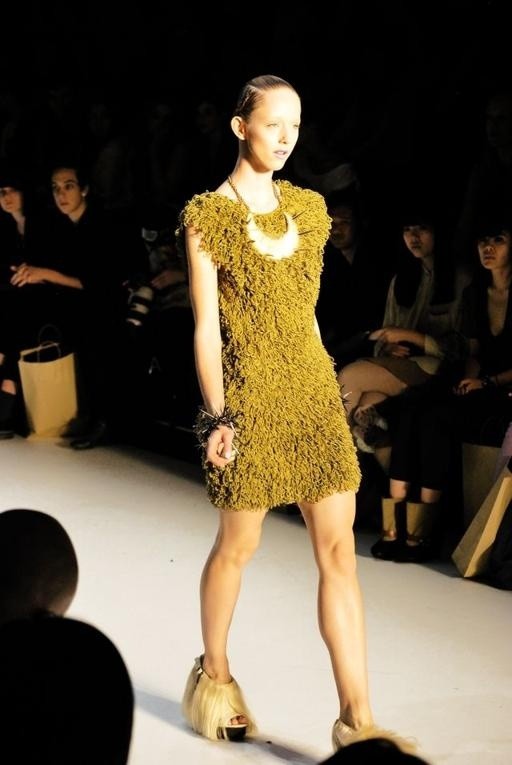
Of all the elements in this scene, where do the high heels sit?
[179,653,259,739]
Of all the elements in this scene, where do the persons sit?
[176,74,414,752]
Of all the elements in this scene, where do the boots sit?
[370,495,443,564]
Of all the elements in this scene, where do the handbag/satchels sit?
[450,454,512,579]
[18,340,78,439]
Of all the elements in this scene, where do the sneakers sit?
[71,420,110,450]
